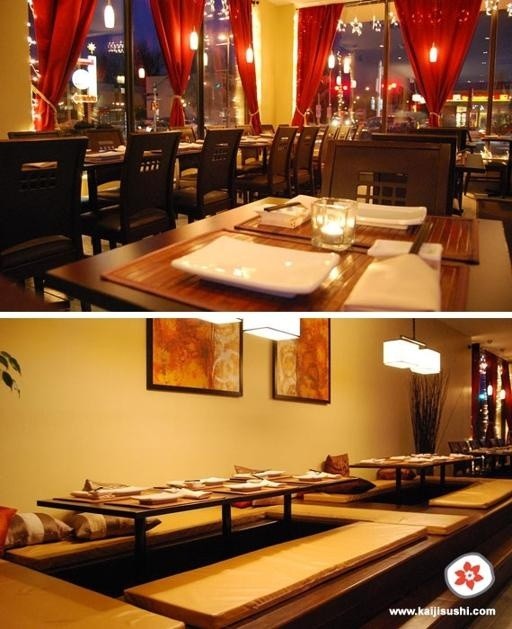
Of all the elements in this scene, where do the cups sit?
[311,197,355,255]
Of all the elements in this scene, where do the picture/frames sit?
[146,318,242,397]
[273,319,332,403]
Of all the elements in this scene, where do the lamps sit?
[328,51,335,69]
[383,318,440,374]
[429,42,437,62]
[246,44,253,63]
[198,318,301,341]
[104,0,115,28]
[189,25,198,50]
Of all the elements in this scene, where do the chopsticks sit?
[410,222,431,259]
[263,201,301,212]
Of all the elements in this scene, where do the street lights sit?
[327,49,354,117]
[216,33,237,125]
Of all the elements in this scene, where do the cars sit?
[329,109,428,141]
[136,115,197,132]
[461,127,509,156]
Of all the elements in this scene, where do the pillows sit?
[314,477,376,494]
[326,454,350,477]
[234,466,285,506]
[231,500,252,509]
[377,468,416,480]
[6,513,75,549]
[0,506,17,558]
[61,511,161,538]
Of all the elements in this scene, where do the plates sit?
[85,131,302,162]
[359,448,475,467]
[172,235,342,300]
[354,204,432,229]
[70,467,338,504]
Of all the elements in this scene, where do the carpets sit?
[476,198,511,258]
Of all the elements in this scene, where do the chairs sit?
[0,123,484,312]
[449,438,504,476]
